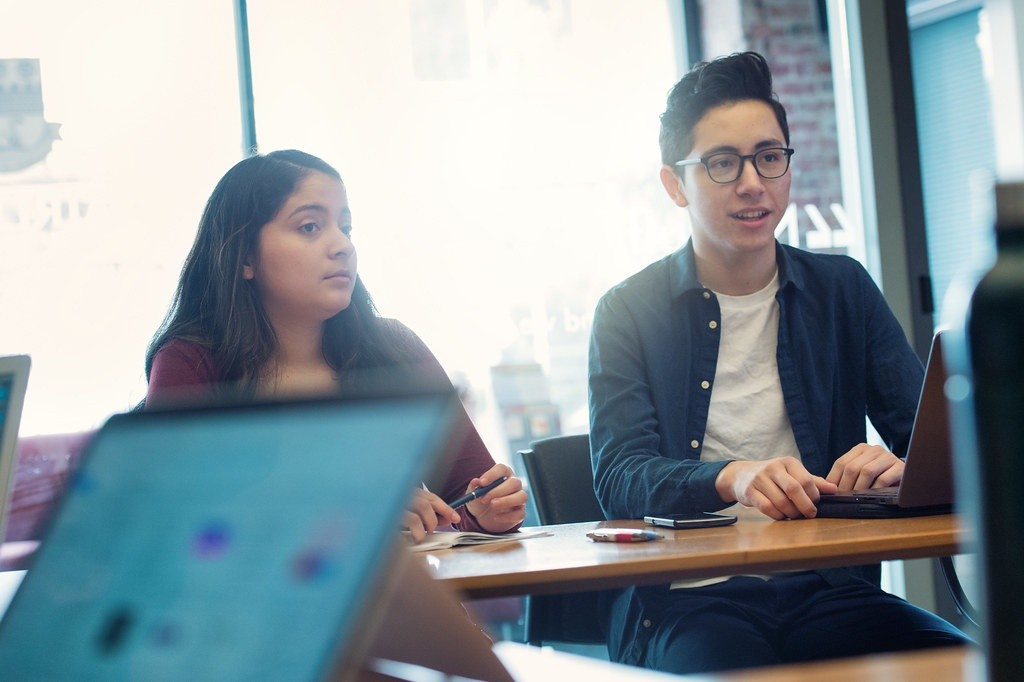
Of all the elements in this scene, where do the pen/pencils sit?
[437,476,508,521]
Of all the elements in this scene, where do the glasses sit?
[674,148,794,183]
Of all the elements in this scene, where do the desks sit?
[0,505,963,603]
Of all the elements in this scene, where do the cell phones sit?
[644,512,738,529]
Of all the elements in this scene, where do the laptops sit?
[0,355,31,538]
[814,331,957,506]
[0,384,458,682]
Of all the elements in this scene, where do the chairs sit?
[520,433,631,648]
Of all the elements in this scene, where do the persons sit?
[132,147,527,547]
[586,51,981,681]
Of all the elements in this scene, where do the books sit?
[400,529,554,553]
